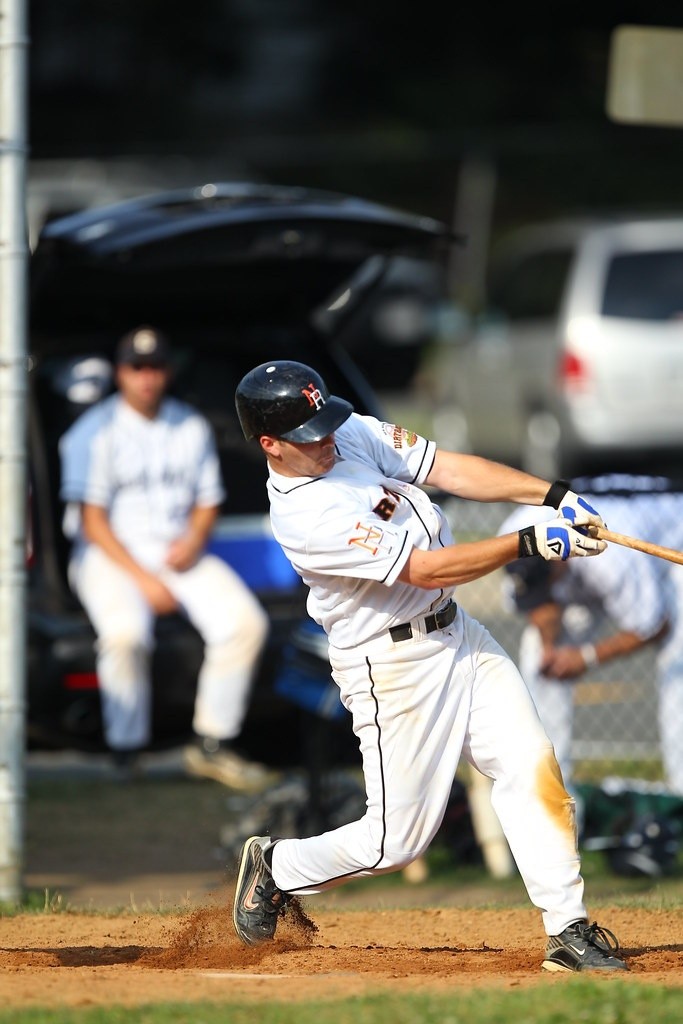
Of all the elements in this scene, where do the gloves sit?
[545,478,605,537]
[518,516,604,564]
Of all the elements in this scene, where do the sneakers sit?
[542,918,628,975]
[231,835,293,948]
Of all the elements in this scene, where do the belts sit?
[387,598,461,639]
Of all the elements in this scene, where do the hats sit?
[119,327,176,369]
[506,554,555,611]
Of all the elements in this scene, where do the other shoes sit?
[104,741,270,792]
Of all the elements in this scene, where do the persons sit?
[232,360,631,978]
[497,494,682,876]
[57,325,271,788]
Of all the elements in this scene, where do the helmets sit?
[235,359,356,445]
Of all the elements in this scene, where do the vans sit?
[418,220,683,483]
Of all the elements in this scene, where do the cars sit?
[28,185,445,389]
